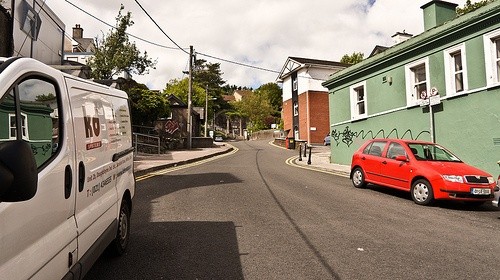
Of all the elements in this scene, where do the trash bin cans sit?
[285,137,290,149]
[288,137,295,149]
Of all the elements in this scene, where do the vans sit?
[1,57,137,280]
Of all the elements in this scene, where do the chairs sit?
[410,148,425,160]
[370,146,382,156]
[388,148,400,158]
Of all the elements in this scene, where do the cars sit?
[350,137,497,206]
[323,130,330,146]
